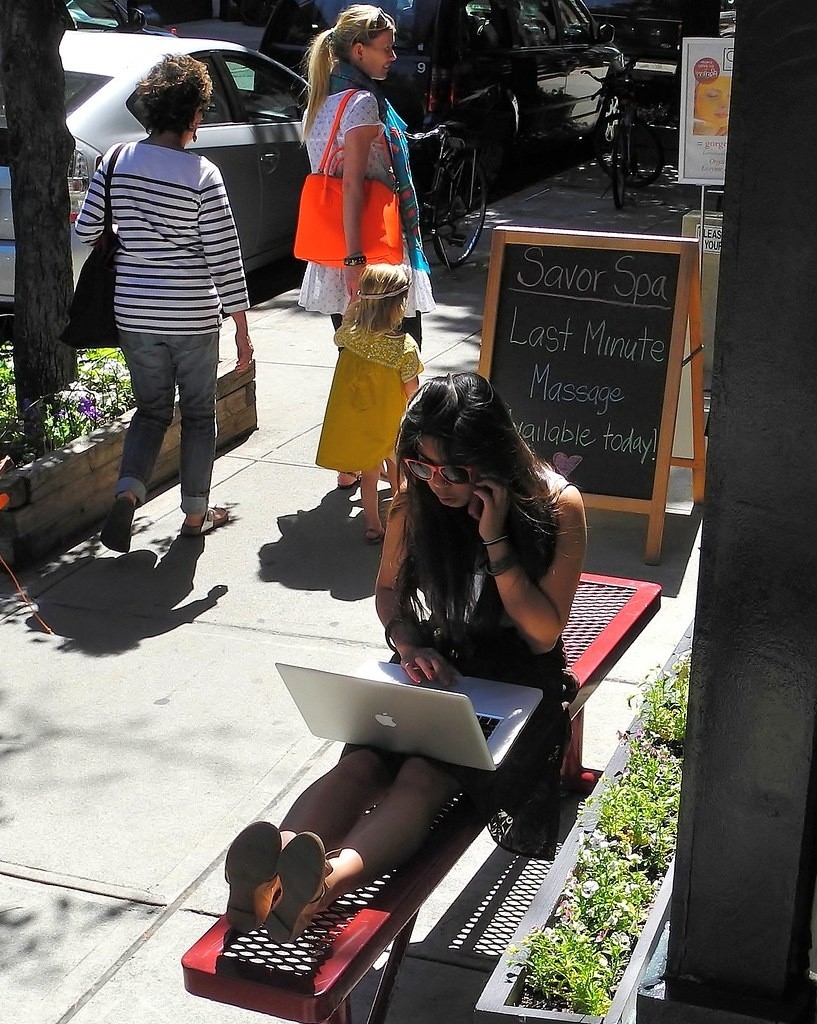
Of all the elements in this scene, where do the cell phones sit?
[468,477,494,520]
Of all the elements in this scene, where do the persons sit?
[73,56,254,553]
[316,263,424,543]
[693,77,731,136]
[297,4,437,488]
[225,372,588,941]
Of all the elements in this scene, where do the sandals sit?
[364,524,386,544]
[337,470,363,490]
[224,821,283,937]
[264,830,343,944]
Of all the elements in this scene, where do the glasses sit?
[402,456,473,486]
[369,7,387,35]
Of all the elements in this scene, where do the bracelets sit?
[485,552,517,576]
[385,612,419,653]
[482,534,509,546]
[344,253,366,266]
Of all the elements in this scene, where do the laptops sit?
[275,659,544,771]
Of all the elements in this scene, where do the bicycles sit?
[403,119,491,280]
[579,50,667,210]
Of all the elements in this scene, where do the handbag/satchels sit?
[293,88,403,273]
[57,141,128,349]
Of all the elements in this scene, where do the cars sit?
[139,0,686,224]
[0,0,311,343]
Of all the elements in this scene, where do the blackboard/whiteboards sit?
[478,224,706,513]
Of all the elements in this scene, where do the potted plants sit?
[472,618,696,1024]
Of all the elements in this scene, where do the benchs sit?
[180,574,662,1024]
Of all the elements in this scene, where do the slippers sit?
[100,496,135,554]
[180,506,228,537]
